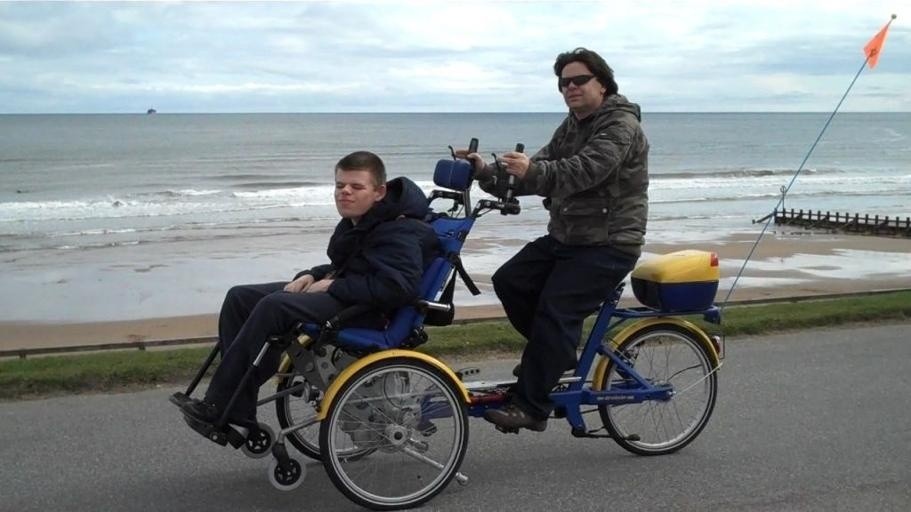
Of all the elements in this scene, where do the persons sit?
[168,151,442,431]
[455,45,654,432]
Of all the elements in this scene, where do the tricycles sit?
[181,137,726,509]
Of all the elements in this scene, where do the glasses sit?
[558,72,596,88]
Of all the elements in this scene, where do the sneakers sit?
[178,397,250,449]
[483,401,550,432]
